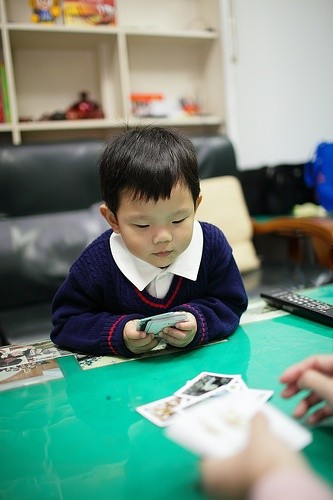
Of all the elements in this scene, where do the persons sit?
[48,125,248,359]
[199,353,333,500]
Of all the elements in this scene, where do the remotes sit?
[259,288,333,328]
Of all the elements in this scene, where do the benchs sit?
[0,135,333,345]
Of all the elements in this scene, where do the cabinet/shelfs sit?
[1,0,231,144]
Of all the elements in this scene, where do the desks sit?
[0,284,333,500]
